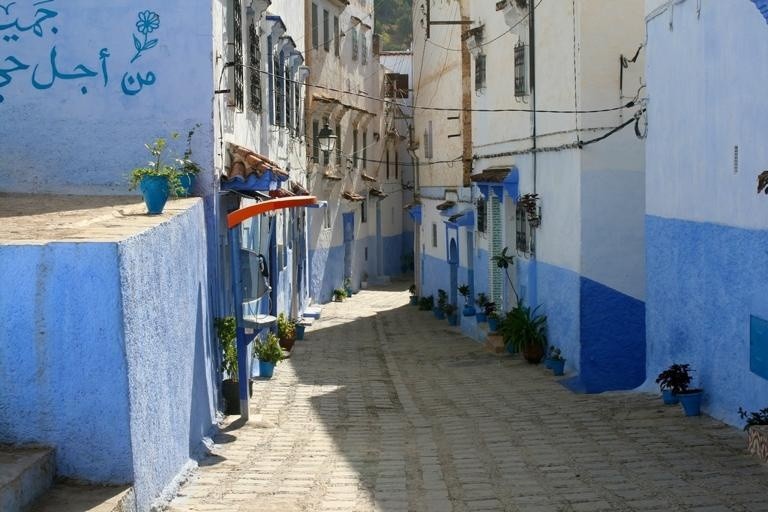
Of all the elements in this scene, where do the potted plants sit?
[333,278,352,302]
[408,248,566,376]
[213,316,253,414]
[128,123,202,214]
[657,364,704,416]
[254,332,282,377]
[278,312,295,351]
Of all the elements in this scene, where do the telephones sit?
[239,247,273,304]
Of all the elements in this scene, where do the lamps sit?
[306,124,338,156]
